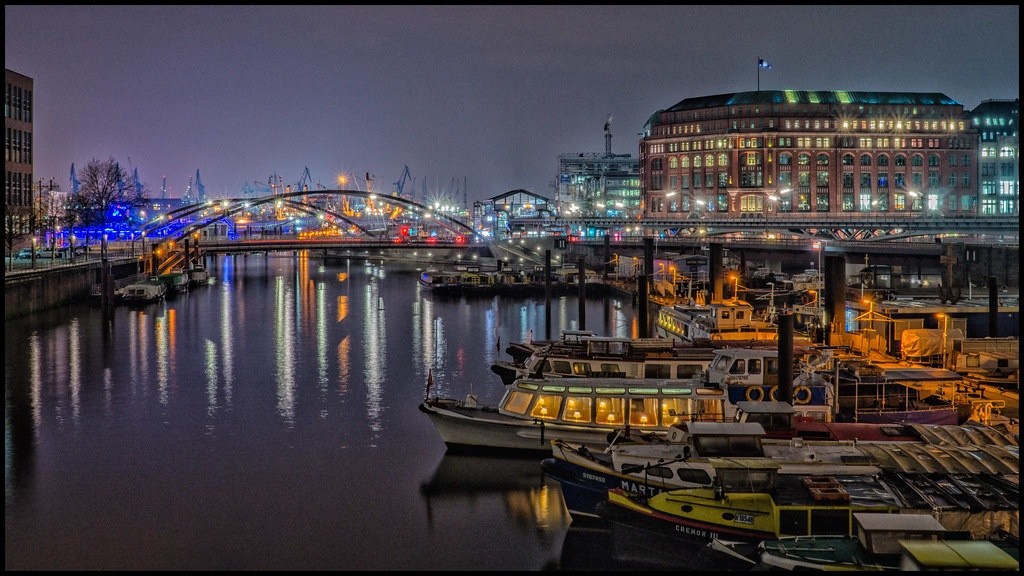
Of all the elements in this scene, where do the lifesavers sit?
[769,385,778,402]
[801,295,809,305]
[773,334,778,340]
[745,385,764,403]
[793,386,812,404]
[712,334,723,341]
[974,403,991,420]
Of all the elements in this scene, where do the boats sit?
[416,254,1018,570]
[419,449,567,539]
[93,264,210,310]
